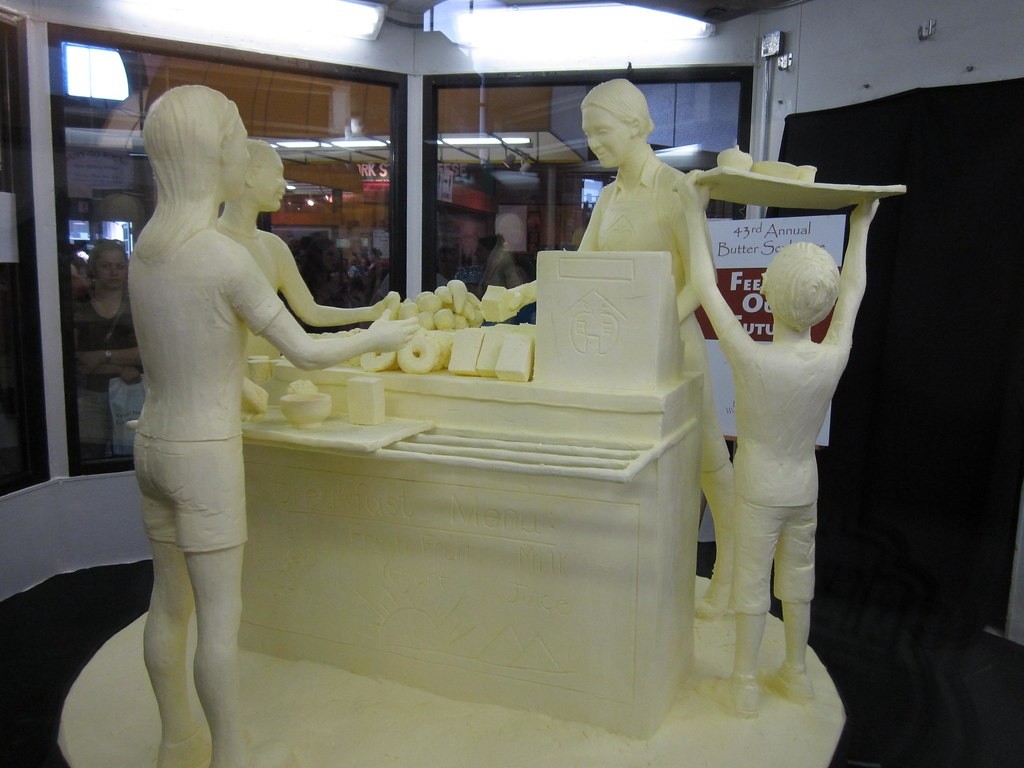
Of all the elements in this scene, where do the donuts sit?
[359,329,454,374]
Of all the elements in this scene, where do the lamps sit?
[519,156,531,175]
[503,148,516,169]
[320,185,329,202]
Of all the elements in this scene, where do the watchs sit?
[104,349,112,363]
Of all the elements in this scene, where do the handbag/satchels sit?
[106,374,144,458]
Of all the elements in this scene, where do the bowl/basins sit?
[716,148,753,172]
[280,393,332,430]
[799,165,817,183]
[753,161,798,180]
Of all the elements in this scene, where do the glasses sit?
[94,239,125,249]
[326,252,333,257]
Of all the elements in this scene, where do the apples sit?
[717,144,753,171]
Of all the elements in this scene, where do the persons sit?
[680,169,880,719]
[437,246,459,287]
[215,138,395,357]
[476,235,520,300]
[478,78,736,620]
[71,239,144,449]
[288,231,389,333]
[126,85,421,768]
